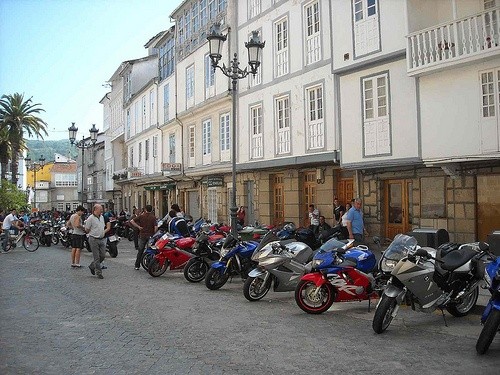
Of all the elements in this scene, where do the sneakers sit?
[71,263,83,268]
[134,267,140,270]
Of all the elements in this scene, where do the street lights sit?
[206,22,266,240]
[24,155,46,217]
[68,122,99,225]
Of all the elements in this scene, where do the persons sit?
[346,197,369,246]
[0,205,184,269]
[236,205,246,230]
[2,209,28,252]
[130,205,158,270]
[66,205,87,269]
[305,198,356,250]
[85,204,107,280]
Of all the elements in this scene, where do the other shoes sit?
[102,264,107,269]
[2,247,7,252]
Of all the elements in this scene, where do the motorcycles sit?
[372,233,490,334]
[243,229,323,302]
[475,230,500,354]
[205,230,291,291]
[37,211,231,285]
[295,237,412,315]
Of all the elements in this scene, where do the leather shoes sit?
[89,266,96,275]
[98,275,104,279]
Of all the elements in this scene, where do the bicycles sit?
[0,223,40,254]
